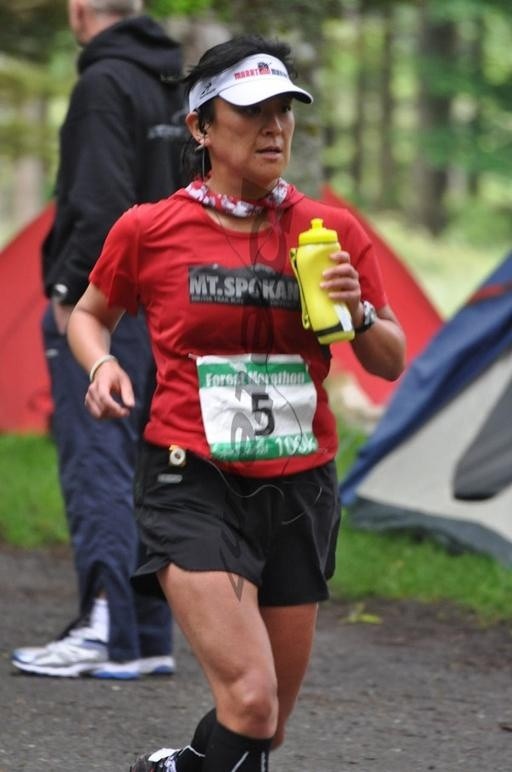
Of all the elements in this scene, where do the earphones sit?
[193,107,206,133]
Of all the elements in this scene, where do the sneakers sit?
[9,623,144,681]
[141,654,177,676]
[128,746,182,770]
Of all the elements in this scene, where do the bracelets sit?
[89,353,119,384]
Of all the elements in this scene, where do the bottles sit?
[290,214,356,347]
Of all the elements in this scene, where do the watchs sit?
[49,285,72,305]
[355,301,377,335]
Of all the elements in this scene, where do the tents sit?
[337,251,512,568]
[0,182,451,436]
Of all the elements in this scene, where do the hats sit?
[187,50,313,114]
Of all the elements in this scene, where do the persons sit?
[8,0,213,681]
[65,37,407,772]
